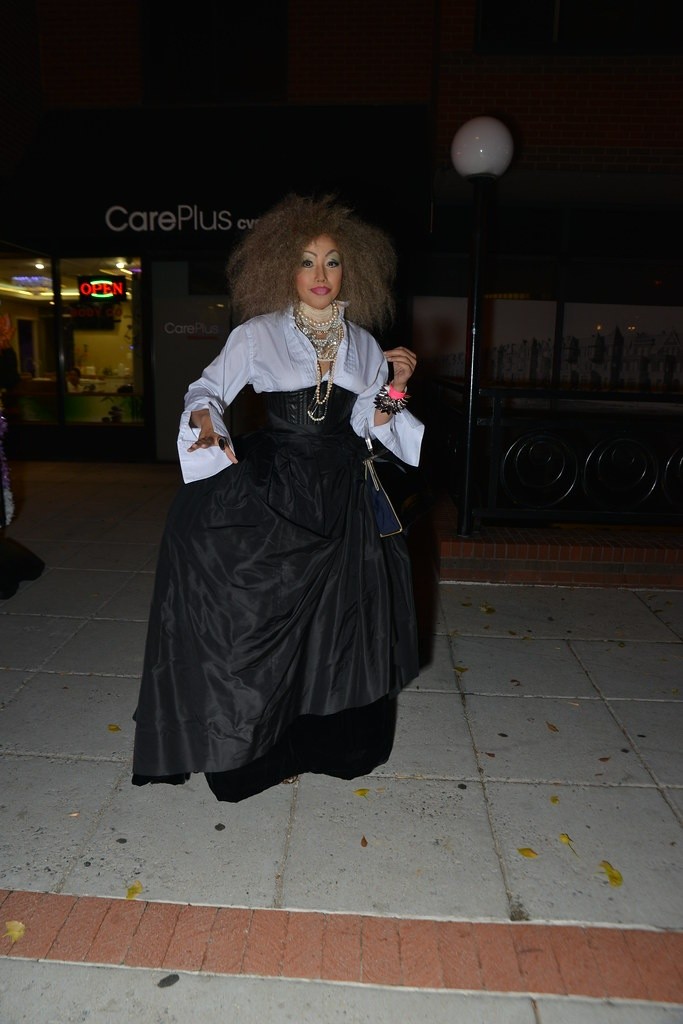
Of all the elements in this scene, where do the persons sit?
[131,197,424,783]
[67,368,86,392]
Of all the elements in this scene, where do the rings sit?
[217,436,226,443]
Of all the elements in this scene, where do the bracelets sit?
[373,380,412,416]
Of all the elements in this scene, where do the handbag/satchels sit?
[359,417,436,536]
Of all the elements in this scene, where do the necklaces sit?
[295,301,344,421]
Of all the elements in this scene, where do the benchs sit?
[15,379,58,394]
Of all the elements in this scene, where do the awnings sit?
[0,106,431,250]
